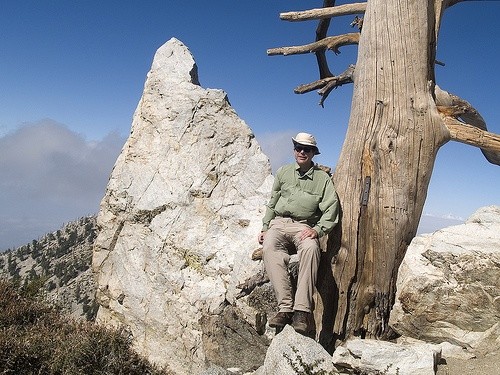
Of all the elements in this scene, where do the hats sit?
[292,133,321,155]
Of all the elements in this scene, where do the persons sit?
[258,133,340,332]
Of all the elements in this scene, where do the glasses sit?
[294,146,313,153]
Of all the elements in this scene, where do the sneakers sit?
[294,311,311,332]
[268,312,293,327]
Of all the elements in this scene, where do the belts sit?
[282,215,316,227]
